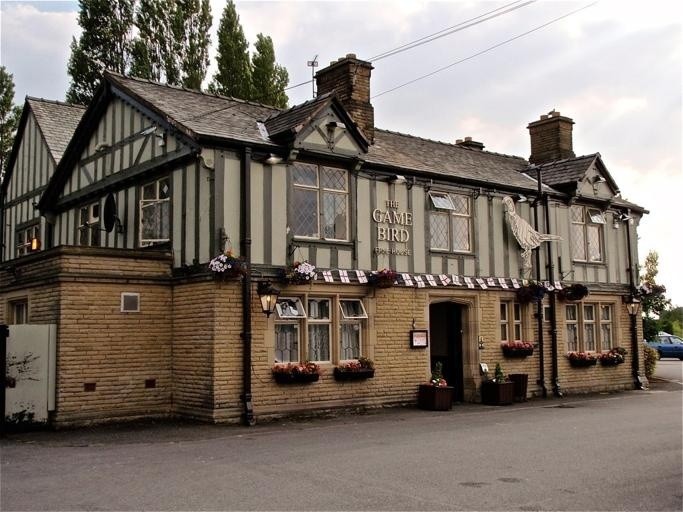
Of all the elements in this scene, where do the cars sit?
[644,335,682,361]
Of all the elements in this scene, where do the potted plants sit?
[418,362,454,411]
[481,362,515,406]
[559,284,589,303]
[516,282,547,305]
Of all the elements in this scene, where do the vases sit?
[569,359,597,368]
[503,348,533,358]
[273,372,319,385]
[334,368,374,381]
[600,358,625,367]
[376,278,396,288]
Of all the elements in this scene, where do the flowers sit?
[283,260,319,283]
[272,358,321,375]
[500,340,533,350]
[335,357,373,371]
[209,253,249,287]
[374,267,395,278]
[567,350,596,361]
[597,347,628,361]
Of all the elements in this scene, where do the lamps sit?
[256,279,280,319]
[622,294,642,318]
